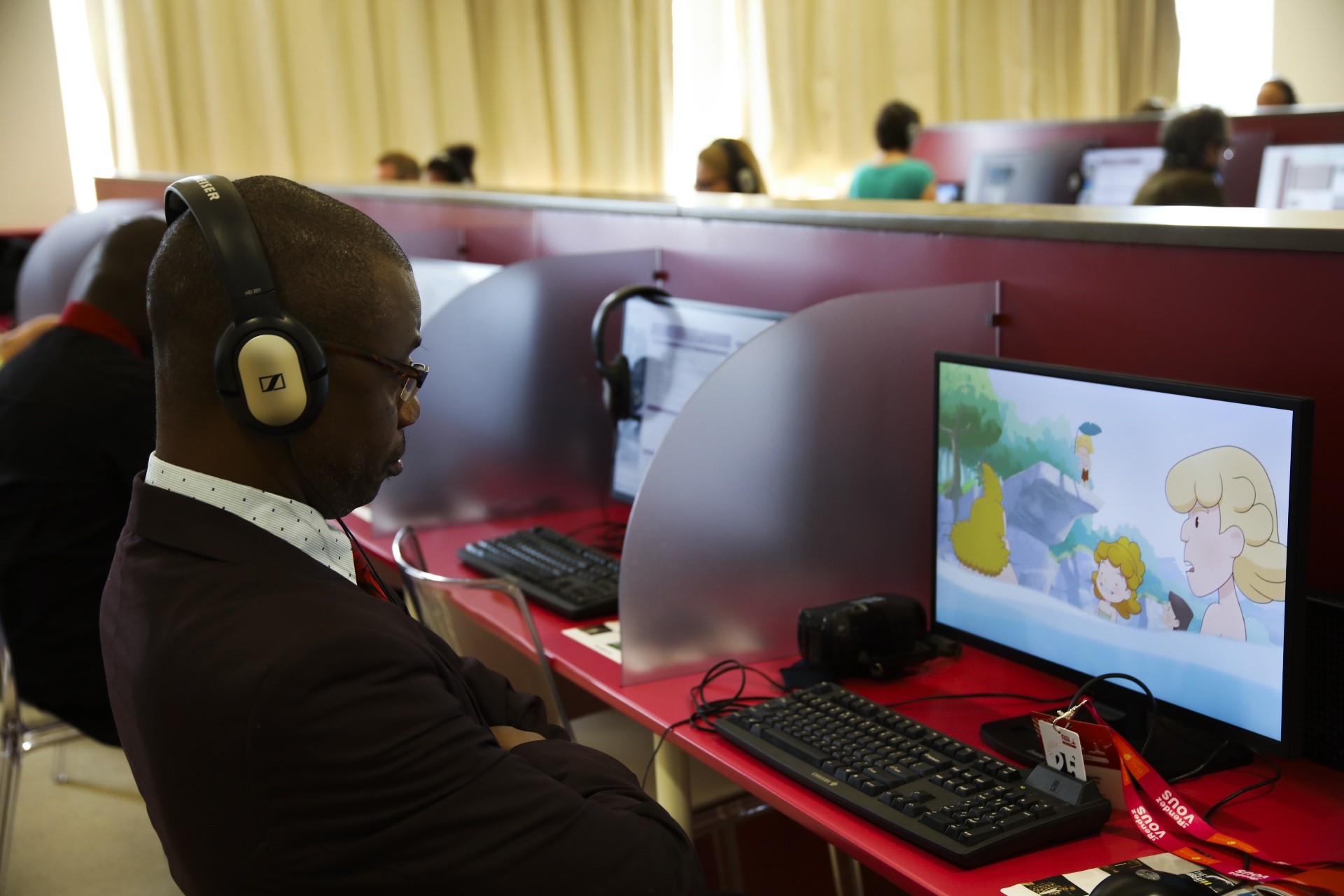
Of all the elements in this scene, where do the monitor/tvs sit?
[929,348,1318,790]
[1253,142,1344,210]
[1067,143,1167,206]
[607,295,799,507]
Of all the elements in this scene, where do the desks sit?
[345,478,1344,896]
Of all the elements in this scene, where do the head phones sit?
[165,175,328,440]
[436,150,472,189]
[712,138,759,193]
[590,285,674,420]
[1065,146,1095,195]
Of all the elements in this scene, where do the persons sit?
[847,98,939,199]
[378,153,422,184]
[1135,103,1239,208]
[693,136,766,193]
[1255,80,1297,108]
[0,218,176,746]
[100,174,700,894]
[423,158,469,184]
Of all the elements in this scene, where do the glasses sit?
[314,339,430,404]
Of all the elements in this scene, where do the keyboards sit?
[715,680,1110,871]
[457,526,619,622]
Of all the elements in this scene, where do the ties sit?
[349,538,390,602]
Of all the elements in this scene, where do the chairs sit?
[392,526,862,896]
[0,637,89,892]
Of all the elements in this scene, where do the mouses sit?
[1088,867,1216,895]
